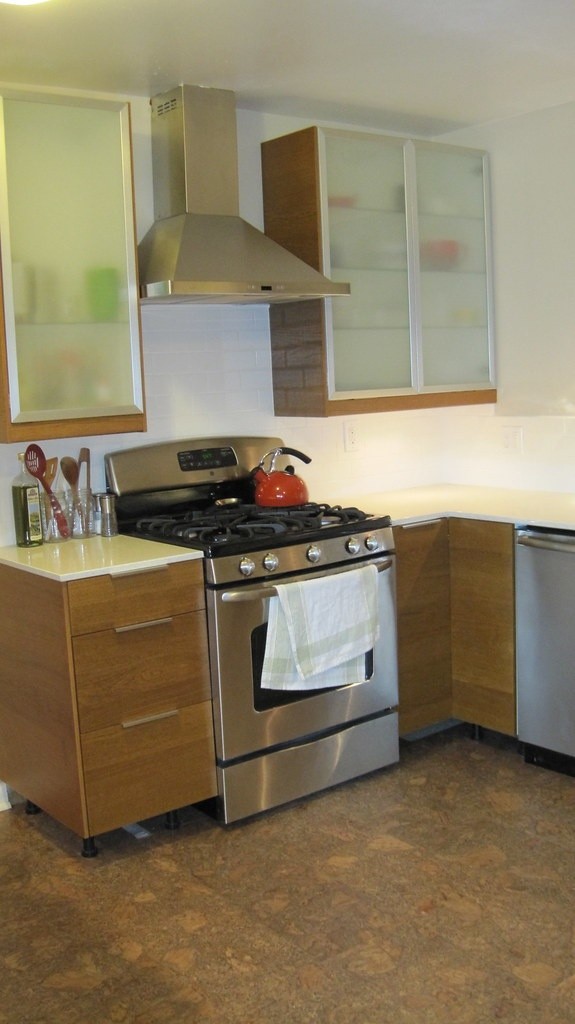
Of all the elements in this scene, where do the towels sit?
[260,564,379,690]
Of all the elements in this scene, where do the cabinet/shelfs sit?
[394,518,516,746]
[1,535,219,858]
[260,124,498,419]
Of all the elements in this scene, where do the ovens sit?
[210,527,401,827]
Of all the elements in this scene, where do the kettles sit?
[250,446,312,507]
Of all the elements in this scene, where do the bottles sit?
[10,452,43,548]
[67,488,95,538]
[40,491,71,543]
[94,493,118,538]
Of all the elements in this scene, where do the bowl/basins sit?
[330,196,354,205]
[87,268,118,320]
[11,262,37,323]
[422,240,460,259]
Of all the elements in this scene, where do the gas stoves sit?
[106,435,392,557]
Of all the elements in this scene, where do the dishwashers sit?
[515,525,575,757]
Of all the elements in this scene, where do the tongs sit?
[71,447,91,535]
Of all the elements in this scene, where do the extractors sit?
[139,214,351,306]
